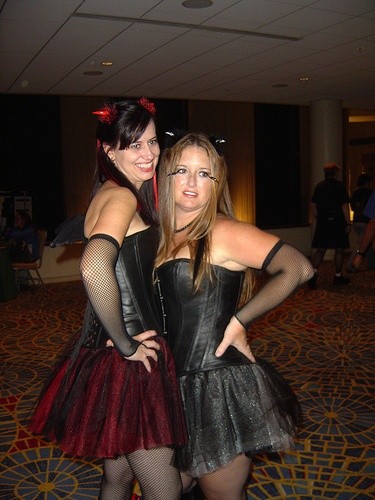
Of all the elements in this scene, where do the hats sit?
[324,163,342,172]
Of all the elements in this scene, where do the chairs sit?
[11,229,48,291]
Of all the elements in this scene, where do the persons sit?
[351,191,375,269]
[0,210,40,301]
[24,99,191,500]
[346,173,375,272]
[153,133,316,500]
[305,162,353,287]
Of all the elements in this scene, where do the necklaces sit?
[174,222,194,232]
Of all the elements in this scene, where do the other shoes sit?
[333,274,351,285]
[309,272,318,289]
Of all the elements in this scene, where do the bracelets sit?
[357,250,364,256]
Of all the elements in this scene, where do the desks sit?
[0,246,18,301]
